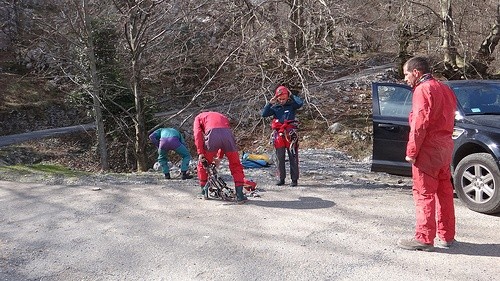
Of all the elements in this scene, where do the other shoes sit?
[276,179,284,186]
[290,180,297,187]
[197,193,208,200]
[182,175,193,180]
[238,195,247,204]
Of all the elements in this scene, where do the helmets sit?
[276,86,288,96]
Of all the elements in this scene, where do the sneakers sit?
[437,237,453,246]
[397,238,434,252]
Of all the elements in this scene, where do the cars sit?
[371,80,500,214]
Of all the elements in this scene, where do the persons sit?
[149,128,193,181]
[261,86,303,186]
[193,111,248,204]
[397,56,457,252]
